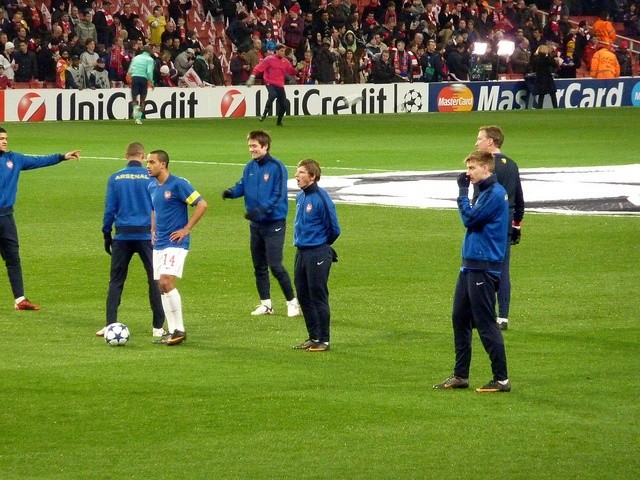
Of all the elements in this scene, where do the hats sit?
[495,2,503,14]
[5,41,15,50]
[290,3,300,14]
[102,0,112,5]
[186,48,195,58]
[72,55,80,60]
[58,39,65,43]
[97,57,105,63]
[160,64,169,74]
[0,54,5,69]
[143,45,151,52]
[113,11,120,19]
[239,11,248,21]
[323,37,331,45]
[252,31,259,35]
[620,39,630,48]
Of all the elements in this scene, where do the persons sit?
[246,44,300,127]
[532,46,559,109]
[544,0,575,79]
[145,151,208,344]
[590,41,620,79]
[476,125,524,330]
[290,158,341,351]
[591,10,616,44]
[222,129,303,318]
[615,0,639,40]
[274,0,544,85]
[575,20,590,69]
[613,41,632,76]
[0,127,81,309]
[138,0,274,87]
[96,143,168,340]
[582,36,609,73]
[128,45,155,126]
[0,1,139,88]
[433,151,513,394]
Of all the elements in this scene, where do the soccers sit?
[105,322,130,346]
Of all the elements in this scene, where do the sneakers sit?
[95,325,107,336]
[433,375,469,388]
[287,298,301,317]
[277,122,283,125]
[250,304,274,315]
[259,115,266,122]
[294,339,320,349]
[475,379,511,392]
[153,331,173,344]
[15,300,40,310]
[166,329,187,346]
[135,119,142,124]
[306,341,330,352]
[133,105,140,119]
[496,317,508,330]
[153,327,167,337]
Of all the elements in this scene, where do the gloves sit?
[457,172,471,188]
[511,220,522,245]
[330,247,339,262]
[247,76,255,88]
[296,61,306,70]
[222,189,233,200]
[152,86,154,91]
[245,208,259,220]
[104,232,113,255]
